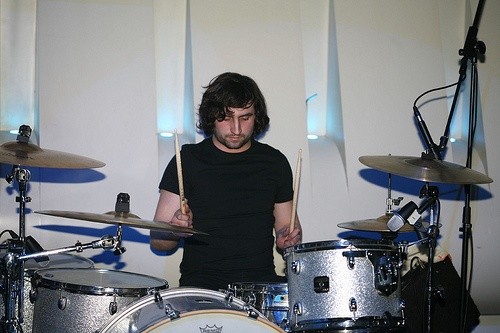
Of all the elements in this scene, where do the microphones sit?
[413,106,441,159]
[387,194,429,232]
[25,236,50,268]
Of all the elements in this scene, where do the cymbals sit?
[0,142,106,169]
[358,155,493,184]
[338,215,443,232]
[34,210,210,237]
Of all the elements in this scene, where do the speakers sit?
[388,258,480,333]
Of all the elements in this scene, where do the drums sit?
[227,281,291,333]
[29,267,170,333]
[284,239,407,332]
[399,237,480,333]
[0,243,96,333]
[95,286,289,333]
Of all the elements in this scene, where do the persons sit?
[150,71,302,290]
